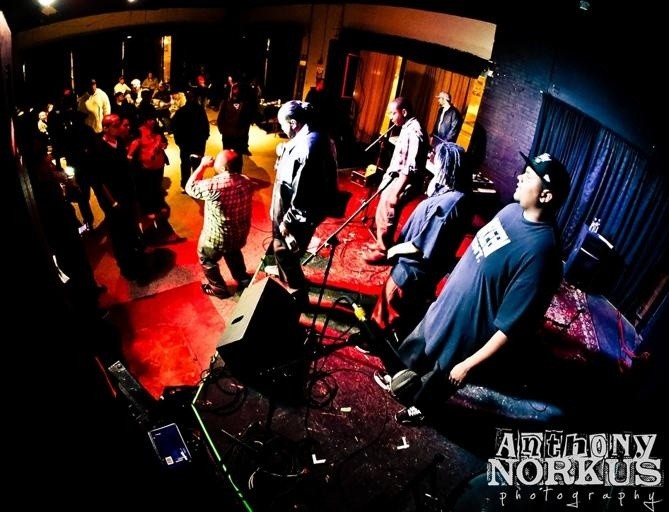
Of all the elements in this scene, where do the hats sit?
[516,147,573,200]
[435,91,450,101]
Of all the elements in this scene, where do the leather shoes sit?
[361,241,391,263]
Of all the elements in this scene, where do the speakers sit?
[564,224,615,296]
[215,276,301,384]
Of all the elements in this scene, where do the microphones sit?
[351,302,422,401]
[190,154,203,160]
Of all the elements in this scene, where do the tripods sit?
[341,122,396,244]
[302,171,398,347]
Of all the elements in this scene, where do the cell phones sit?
[147,422,192,468]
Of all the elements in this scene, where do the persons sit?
[21,66,261,297]
[429,91,462,146]
[267,99,335,307]
[304,79,329,106]
[372,153,574,425]
[358,143,472,354]
[362,96,426,265]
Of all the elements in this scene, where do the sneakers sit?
[373,369,402,401]
[395,403,427,425]
[200,283,229,300]
